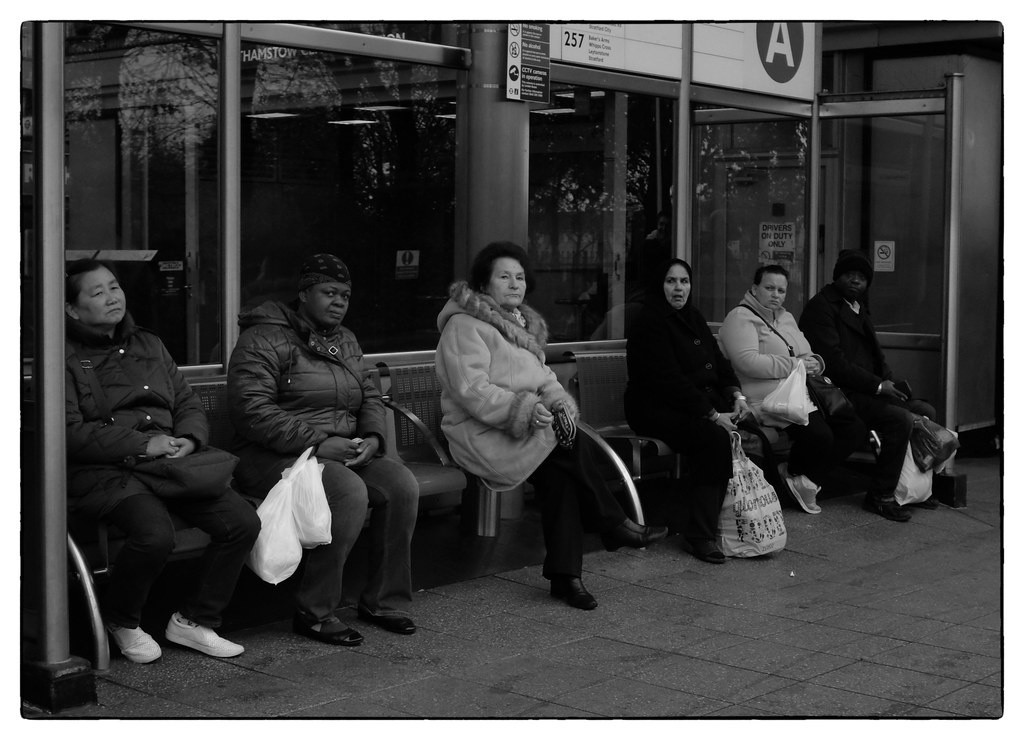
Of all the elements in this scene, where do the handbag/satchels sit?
[243,446,315,585]
[894,411,962,506]
[552,403,577,452]
[130,442,239,500]
[714,427,788,558]
[760,356,811,427]
[239,441,332,550]
[806,373,855,420]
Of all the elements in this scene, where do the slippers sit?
[684,540,726,563]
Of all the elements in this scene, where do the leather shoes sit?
[357,600,417,635]
[550,572,597,611]
[602,516,669,552]
[292,608,365,646]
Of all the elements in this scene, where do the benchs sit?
[21,361,500,586]
[563,347,788,491]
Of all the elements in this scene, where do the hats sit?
[833,247,873,289]
[294,252,351,290]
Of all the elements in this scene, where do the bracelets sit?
[714,413,720,424]
[736,395,748,401]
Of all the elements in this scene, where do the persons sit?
[64,258,261,665]
[435,240,667,609]
[797,249,937,520]
[227,254,420,647]
[716,266,864,514]
[624,258,788,563]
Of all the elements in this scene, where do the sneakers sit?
[165,612,245,657]
[863,490,913,521]
[786,474,822,514]
[102,621,162,663]
[915,495,939,509]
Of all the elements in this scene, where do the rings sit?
[535,420,539,424]
